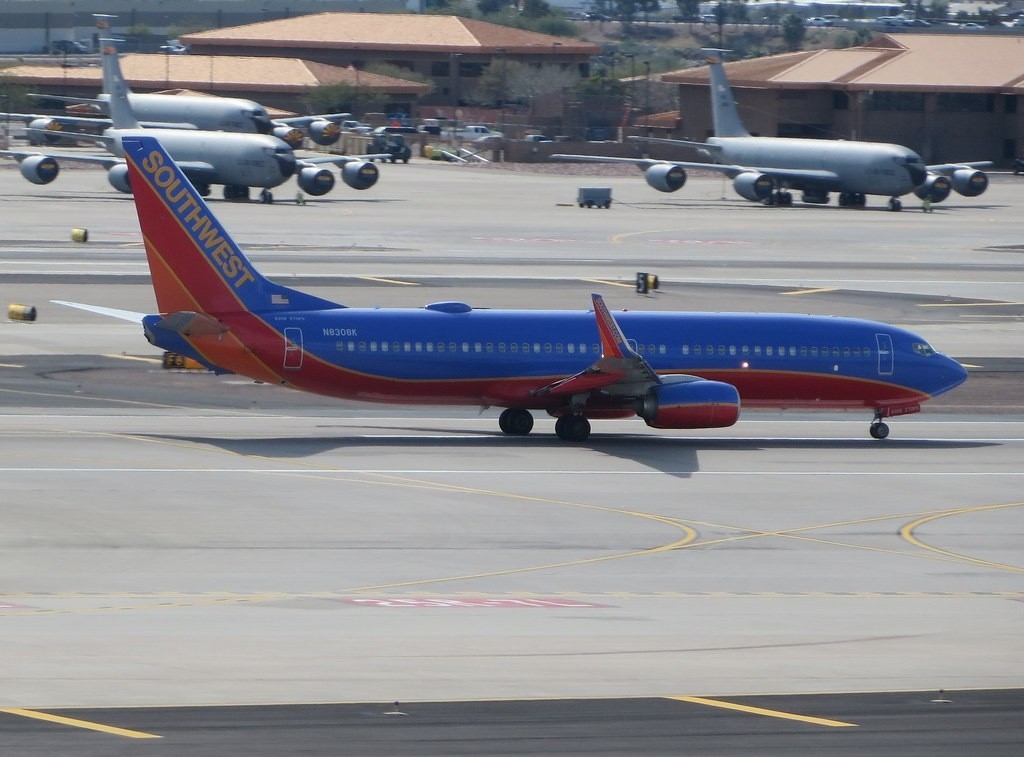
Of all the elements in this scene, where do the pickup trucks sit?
[44,42,88,53]
[441,124,506,146]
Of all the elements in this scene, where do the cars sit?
[807,17,832,26]
[905,20,932,28]
[338,120,368,133]
[368,134,411,163]
[374,124,423,137]
[1010,156,1024,175]
[960,23,985,30]
[588,13,612,23]
[702,15,719,24]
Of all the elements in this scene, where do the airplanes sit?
[24,14,352,149]
[49,137,973,440]
[549,48,996,213]
[0,37,396,207]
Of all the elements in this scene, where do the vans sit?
[524,135,551,143]
